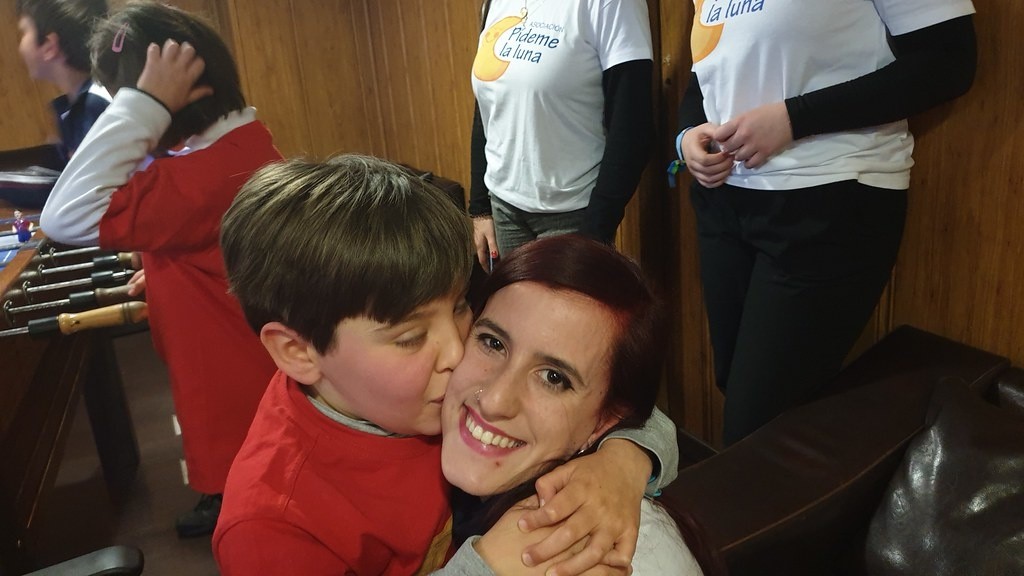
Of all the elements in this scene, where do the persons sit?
[439,234,704,576]
[211,153,679,576]
[15,0,286,542]
[468,1,656,272]
[674,1,977,448]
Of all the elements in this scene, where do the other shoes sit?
[177,493,222,538]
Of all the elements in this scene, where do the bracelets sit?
[667,126,693,174]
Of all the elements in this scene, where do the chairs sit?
[656,322,1024,575]
